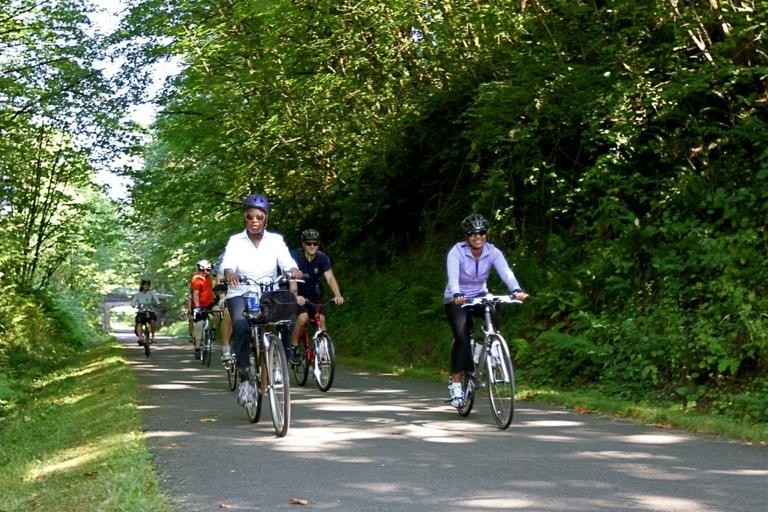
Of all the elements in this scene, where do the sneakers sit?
[221,354,232,370]
[444,381,466,408]
[289,343,301,365]
[195,348,201,359]
[272,369,284,389]
[238,380,250,407]
[209,327,217,340]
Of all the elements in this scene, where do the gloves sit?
[193,306,205,323]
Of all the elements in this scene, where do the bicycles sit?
[210,277,243,392]
[187,305,224,364]
[444,287,521,429]
[290,292,349,393]
[210,268,311,430]
[130,301,162,356]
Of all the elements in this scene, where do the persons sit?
[219,193,309,406]
[287,228,345,364]
[212,251,242,361]
[442,214,530,409]
[128,250,223,360]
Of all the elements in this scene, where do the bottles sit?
[246,281,259,318]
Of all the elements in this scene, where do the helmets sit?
[243,193,271,215]
[301,229,320,243]
[461,214,489,234]
[196,259,212,270]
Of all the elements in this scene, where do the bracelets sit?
[453,293,465,298]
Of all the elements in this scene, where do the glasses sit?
[246,214,264,220]
[466,230,487,237]
[305,242,318,246]
